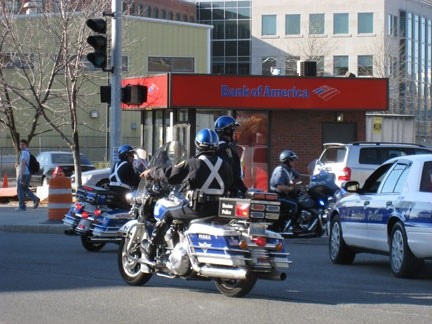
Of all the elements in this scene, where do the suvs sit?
[312,141,432,195]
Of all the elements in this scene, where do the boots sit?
[140,213,171,261]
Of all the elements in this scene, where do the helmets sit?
[195,129,219,150]
[280,150,296,162]
[118,145,134,158]
[214,116,236,135]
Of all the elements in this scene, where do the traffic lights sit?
[122,84,147,105]
[86,19,107,68]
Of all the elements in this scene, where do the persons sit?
[270,150,310,233]
[108,145,141,202]
[15,139,40,211]
[139,128,234,262]
[214,116,248,199]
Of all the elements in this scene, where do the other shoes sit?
[33,198,40,209]
[16,208,26,211]
[293,227,303,233]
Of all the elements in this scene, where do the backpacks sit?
[22,150,39,173]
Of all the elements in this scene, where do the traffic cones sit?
[3,173,8,188]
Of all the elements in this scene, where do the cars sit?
[326,154,432,278]
[236,144,268,189]
[70,158,146,191]
[29,151,95,187]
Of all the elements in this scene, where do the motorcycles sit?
[117,142,293,298]
[61,186,134,252]
[267,160,344,238]
